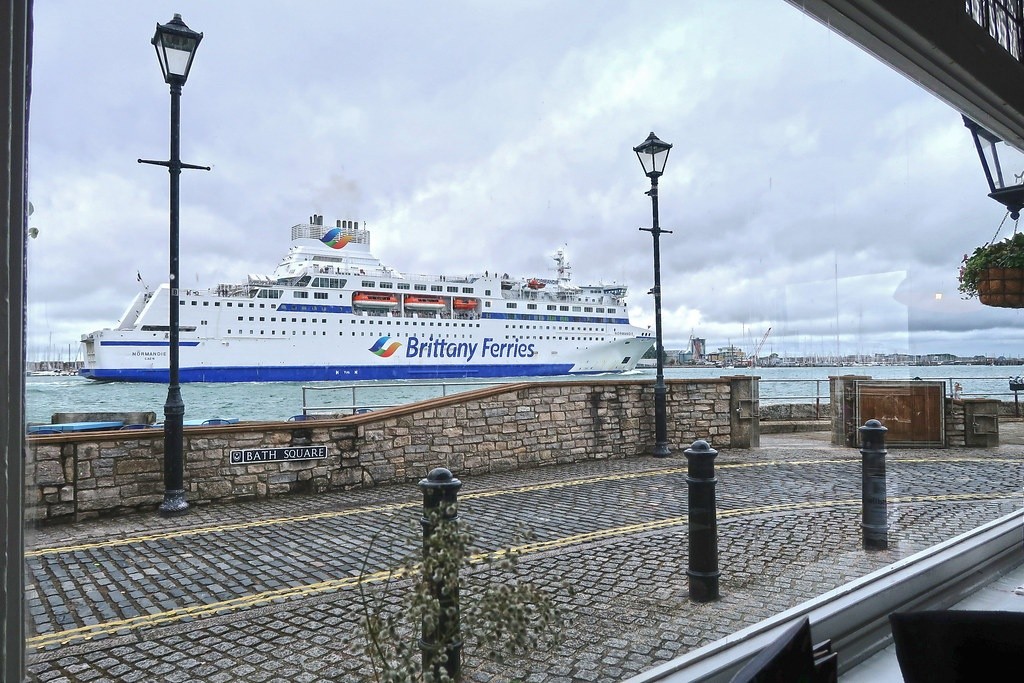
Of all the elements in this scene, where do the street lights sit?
[634,131,678,457]
[136,12,212,514]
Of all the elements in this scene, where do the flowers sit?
[956,231,1024,301]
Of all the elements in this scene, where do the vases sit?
[976,265,1024,308]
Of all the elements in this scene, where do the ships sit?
[75,210,658,371]
